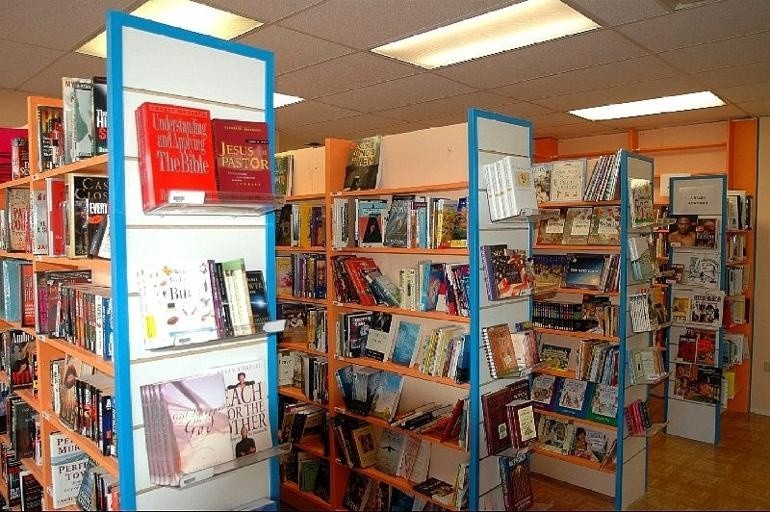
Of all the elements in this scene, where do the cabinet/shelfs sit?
[274,106,539,511]
[533,119,752,511]
[1,9,283,511]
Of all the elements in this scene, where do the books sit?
[140,356,270,488]
[137,257,270,349]
[275,136,543,512]
[531,152,622,470]
[135,103,274,211]
[626,176,666,433]
[3,74,123,512]
[666,190,753,409]
